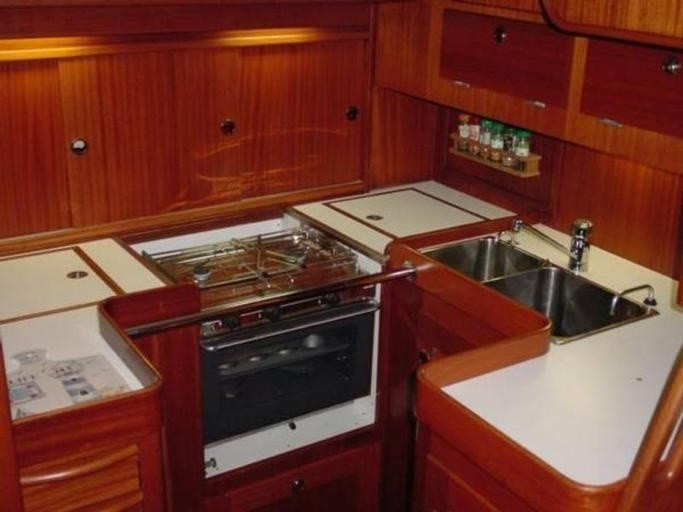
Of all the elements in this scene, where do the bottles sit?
[457,114,467,153]
[501,126,518,167]
[489,123,505,161]
[479,119,492,160]
[466,117,479,153]
[516,131,531,154]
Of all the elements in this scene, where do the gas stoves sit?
[148,223,360,301]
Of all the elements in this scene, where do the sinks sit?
[424,235,541,283]
[483,265,649,338]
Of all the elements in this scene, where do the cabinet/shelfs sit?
[178,26,373,236]
[373,0,683,177]
[389,277,683,512]
[0,27,177,257]
[208,426,388,510]
[0,282,206,512]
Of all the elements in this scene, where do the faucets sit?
[608,283,657,318]
[567,217,596,273]
[496,219,523,246]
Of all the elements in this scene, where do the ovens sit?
[196,302,381,482]
